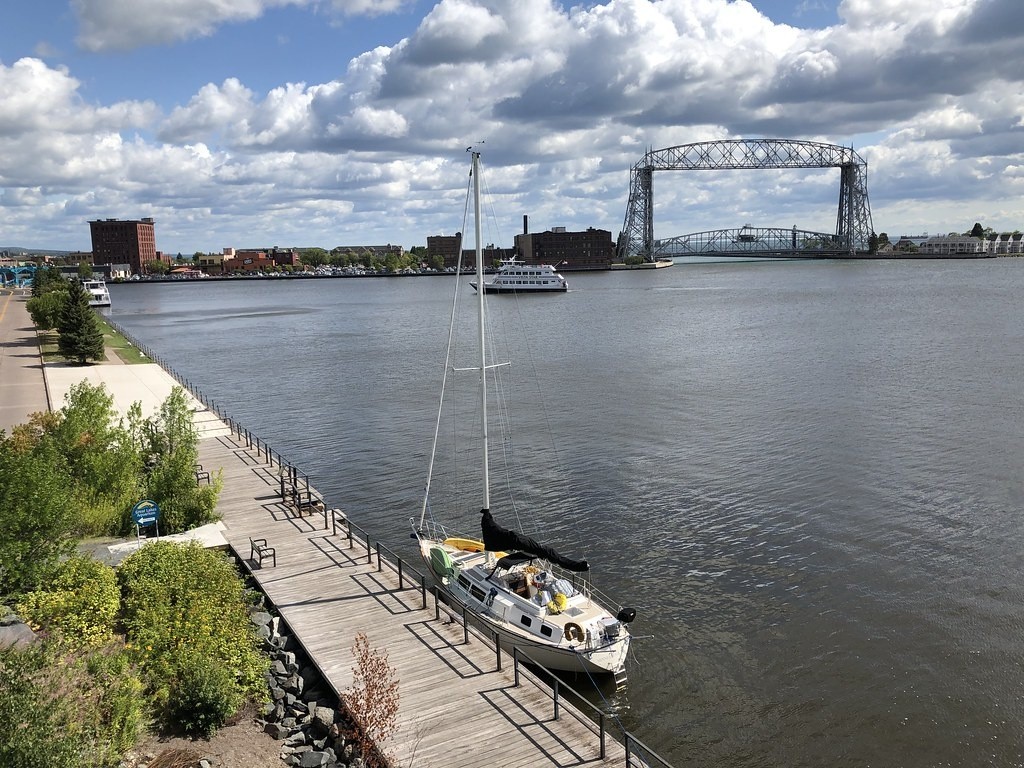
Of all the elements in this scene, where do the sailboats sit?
[404,148,657,686]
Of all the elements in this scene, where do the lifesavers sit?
[564,622,585,642]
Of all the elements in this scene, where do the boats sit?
[468,254,569,294]
[80,279,111,307]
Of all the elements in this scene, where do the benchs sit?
[191,465,210,486]
[249,536,276,569]
